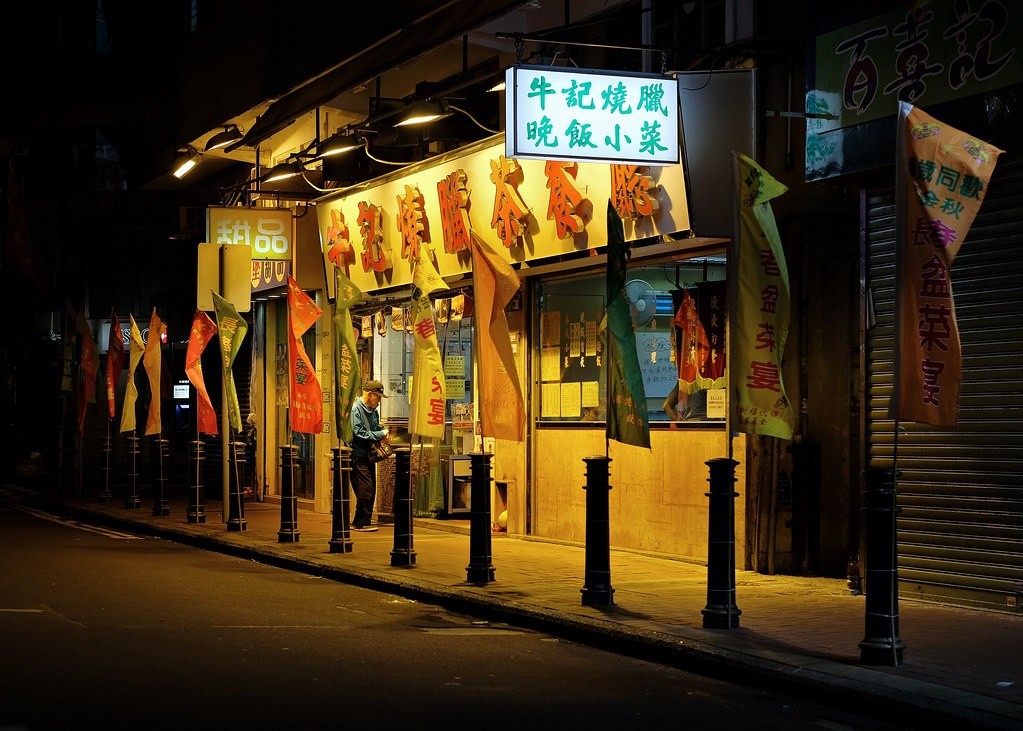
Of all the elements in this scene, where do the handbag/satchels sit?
[367,442,394,463]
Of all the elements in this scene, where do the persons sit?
[348,379,389,531]
[662,381,723,421]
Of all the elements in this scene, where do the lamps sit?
[313,136,365,159]
[485,75,506,92]
[263,161,302,184]
[394,103,454,128]
[203,123,243,152]
[167,146,203,179]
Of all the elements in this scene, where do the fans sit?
[624,279,657,330]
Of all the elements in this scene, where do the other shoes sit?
[354,524,378,531]
[350,524,354,529]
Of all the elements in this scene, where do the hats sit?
[363,380,388,398]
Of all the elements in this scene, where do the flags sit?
[408,243,451,440]
[726,153,795,440]
[77,324,97,432]
[332,267,362,442]
[470,229,526,442]
[212,291,248,434]
[185,310,218,438]
[887,101,1007,430]
[286,274,323,434]
[105,312,126,418]
[606,199,651,449]
[143,312,167,437]
[119,317,145,432]
